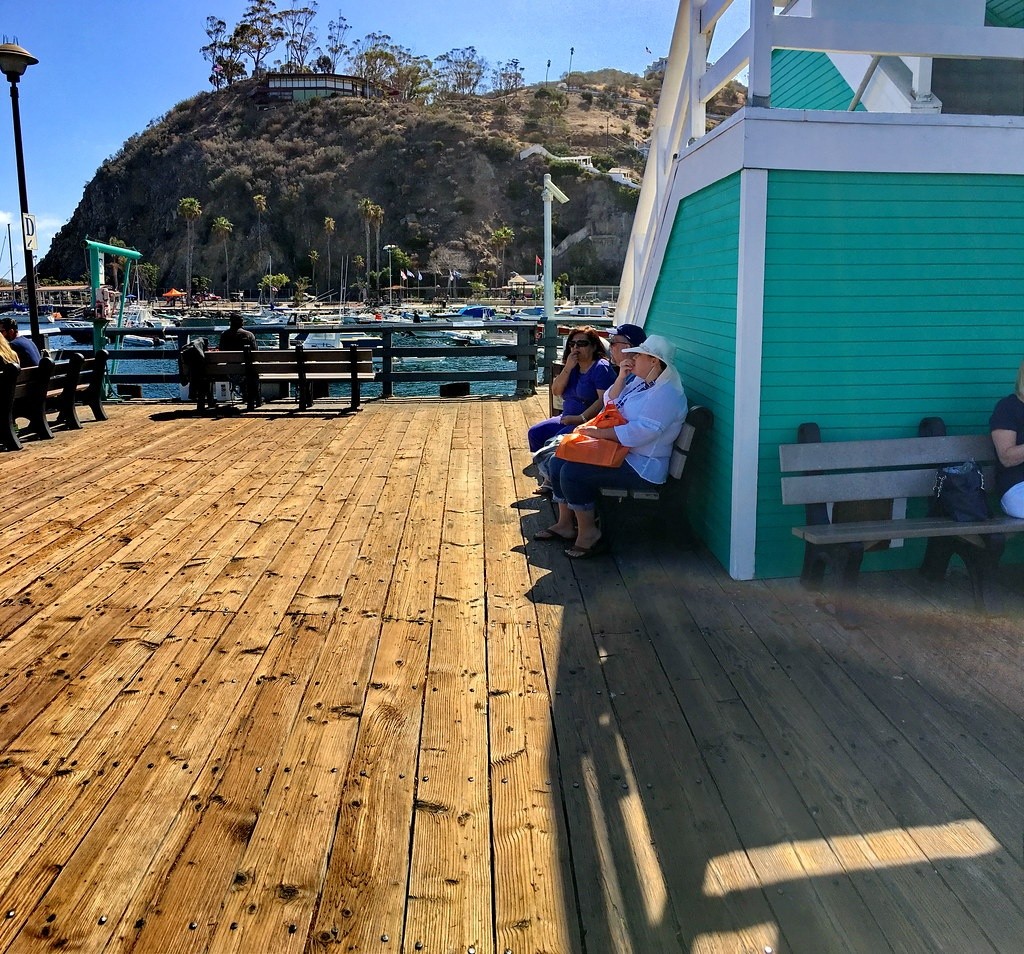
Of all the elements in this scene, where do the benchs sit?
[779,418,1024,630]
[598,399,713,565]
[0,350,109,450]
[192,338,375,417]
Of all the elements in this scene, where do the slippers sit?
[533,529,576,543]
[564,544,607,559]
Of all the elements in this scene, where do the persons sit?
[988,358,1023,523]
[0,316,42,371]
[307,308,350,323]
[418,308,432,314]
[0,270,299,328]
[412,309,420,323]
[351,308,386,320]
[556,308,560,312]
[386,309,403,318]
[220,313,258,403]
[574,298,582,305]
[0,330,21,371]
[446,308,461,314]
[526,325,620,493]
[533,334,689,558]
[609,309,613,313]
[509,293,527,307]
[578,310,581,314]
[585,324,648,423]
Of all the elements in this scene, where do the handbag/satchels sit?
[555,400,632,467]
[933,460,993,521]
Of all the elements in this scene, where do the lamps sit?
[0,34,39,81]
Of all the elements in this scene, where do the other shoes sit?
[533,479,553,494]
[258,397,267,405]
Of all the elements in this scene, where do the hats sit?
[606,324,647,346]
[621,335,676,366]
[230,312,244,321]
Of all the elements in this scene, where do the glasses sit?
[568,340,592,347]
[0,330,6,334]
[611,337,629,345]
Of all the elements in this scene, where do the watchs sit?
[580,413,588,423]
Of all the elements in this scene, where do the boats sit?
[1,284,618,354]
